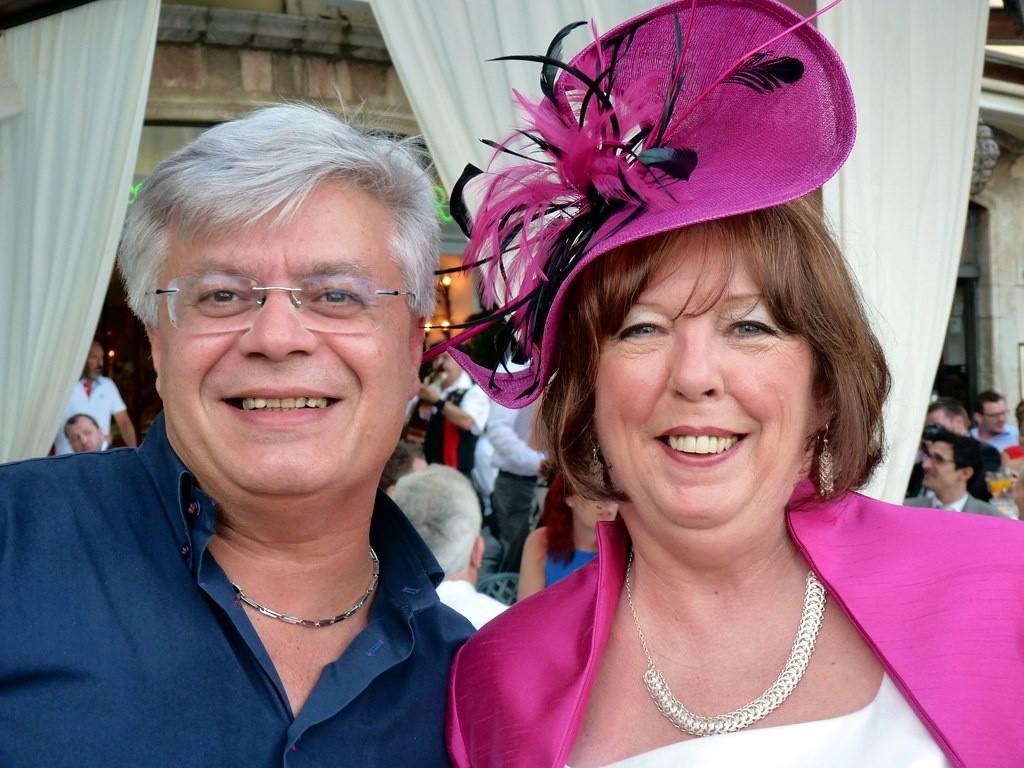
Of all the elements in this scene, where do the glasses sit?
[923,449,955,466]
[979,409,1011,418]
[145,273,417,334]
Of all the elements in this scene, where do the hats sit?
[417,0,858,409]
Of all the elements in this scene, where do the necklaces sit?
[230,538,380,629]
[625,544,826,738]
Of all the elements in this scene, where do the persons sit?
[901,388,1024,518]
[0,101,480,768]
[447,150,1024,767]
[385,311,619,632]
[53,342,138,458]
